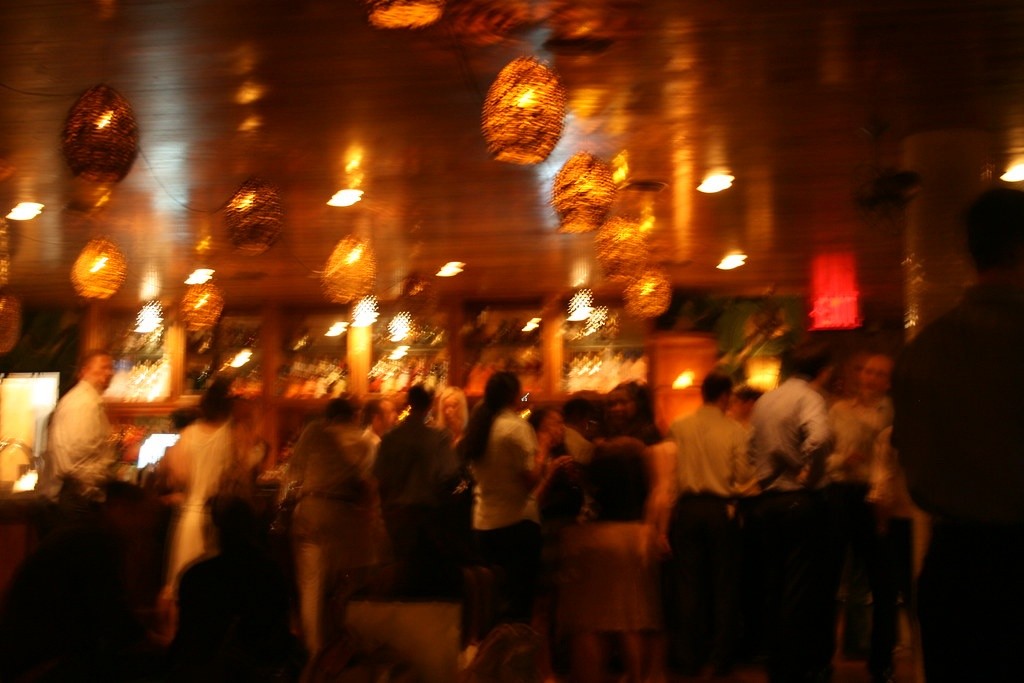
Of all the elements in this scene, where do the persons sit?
[892,189,1024,683]
[0,340,930,683]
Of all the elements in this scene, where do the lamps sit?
[60,0,676,367]
